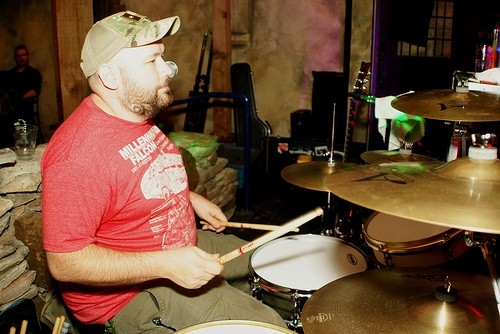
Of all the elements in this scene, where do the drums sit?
[361,211,474,269]
[248,233,371,330]
[170,319,302,334]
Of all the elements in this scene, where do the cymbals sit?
[325,157,500,235]
[280,160,359,193]
[360,150,444,164]
[302,265,500,334]
[391,88,500,122]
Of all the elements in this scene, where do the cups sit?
[13,124,37,160]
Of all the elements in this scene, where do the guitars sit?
[342,60,372,163]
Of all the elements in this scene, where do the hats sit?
[80,10,181,79]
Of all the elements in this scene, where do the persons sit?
[41,9,288,334]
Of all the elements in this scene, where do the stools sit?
[40,294,104,334]
